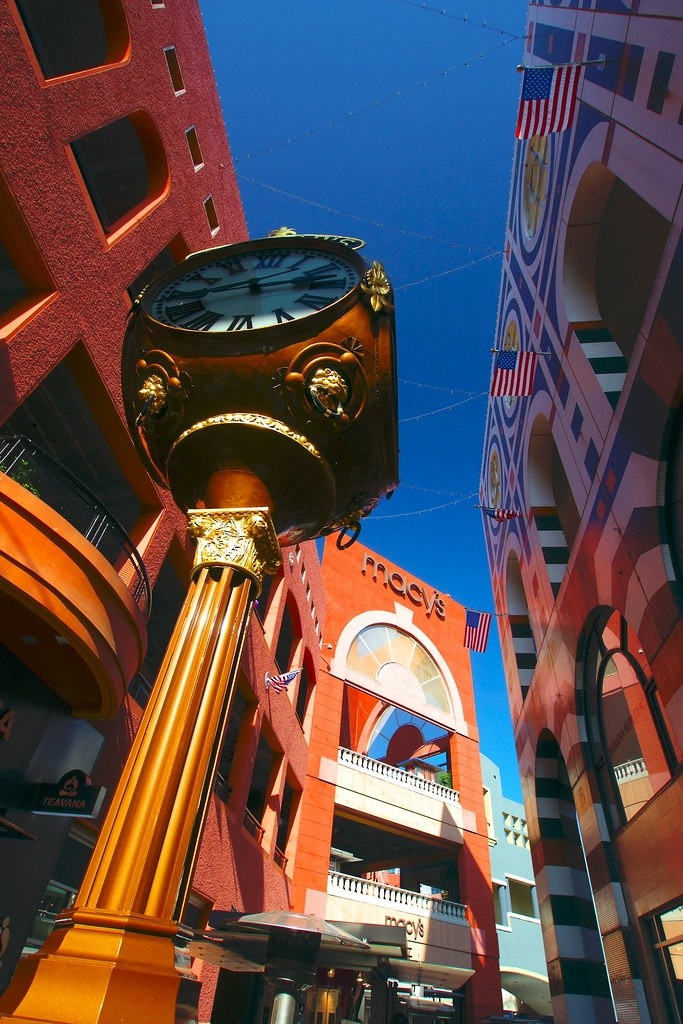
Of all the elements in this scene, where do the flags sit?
[482,506,523,521]
[515,62,581,139]
[489,351,537,397]
[463,609,492,652]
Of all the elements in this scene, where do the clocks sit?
[148,232,366,343]
